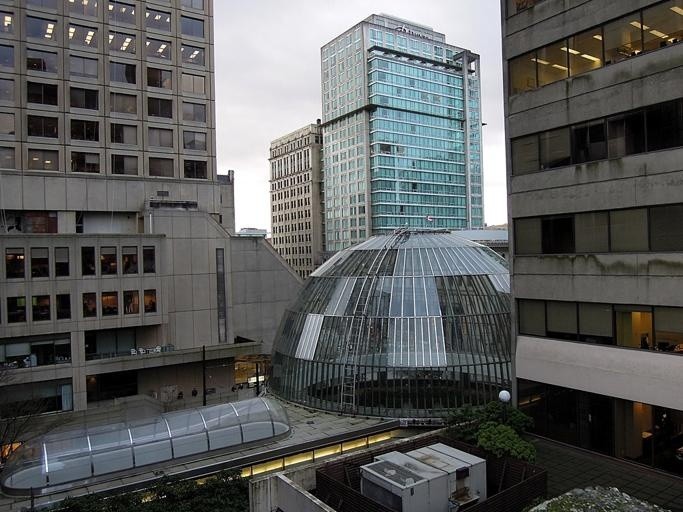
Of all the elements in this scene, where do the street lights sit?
[498,389,510,424]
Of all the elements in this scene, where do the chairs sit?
[130,345,161,356]
